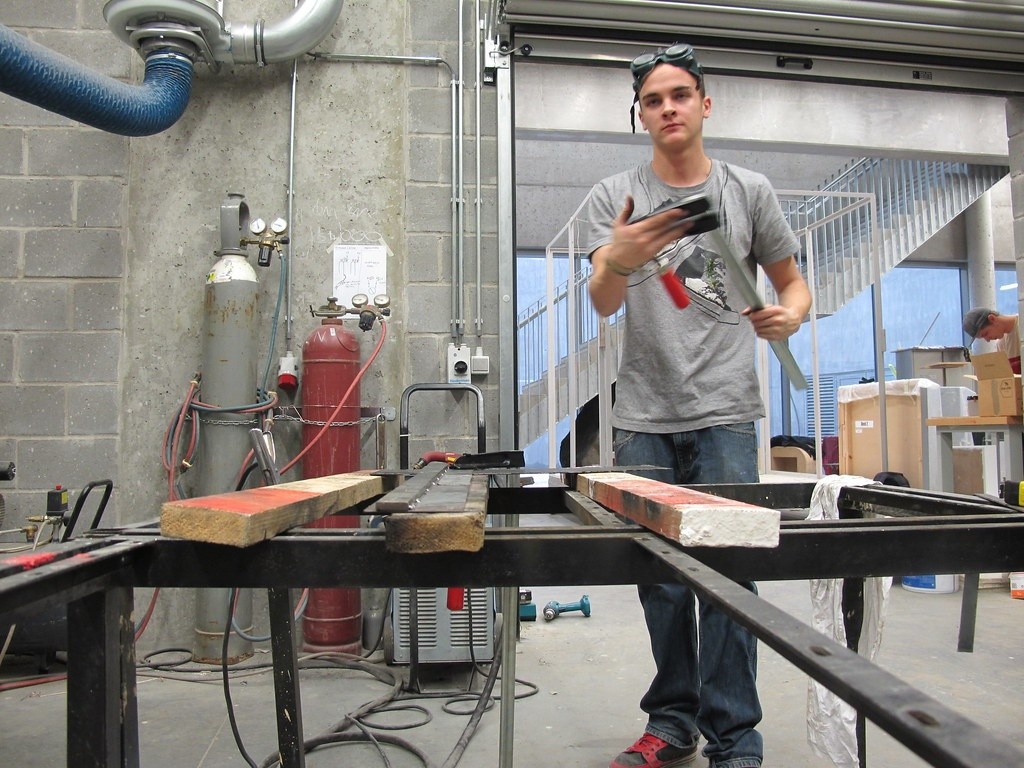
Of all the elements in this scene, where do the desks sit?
[925,417,1024,500]
[920,362,967,386]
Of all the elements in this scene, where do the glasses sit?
[630,44,694,79]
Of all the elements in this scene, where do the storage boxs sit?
[1010,574,1024,599]
[837,378,940,488]
[969,350,1024,417]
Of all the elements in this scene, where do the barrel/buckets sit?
[901,574,961,594]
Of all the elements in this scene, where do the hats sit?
[963,307,991,347]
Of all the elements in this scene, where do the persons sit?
[587,44,815,768]
[963,308,1021,374]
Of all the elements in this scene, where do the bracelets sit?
[606,257,633,277]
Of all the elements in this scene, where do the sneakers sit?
[609,732,697,768]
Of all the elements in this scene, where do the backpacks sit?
[872,472,910,488]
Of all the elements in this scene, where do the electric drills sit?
[543,595,591,621]
